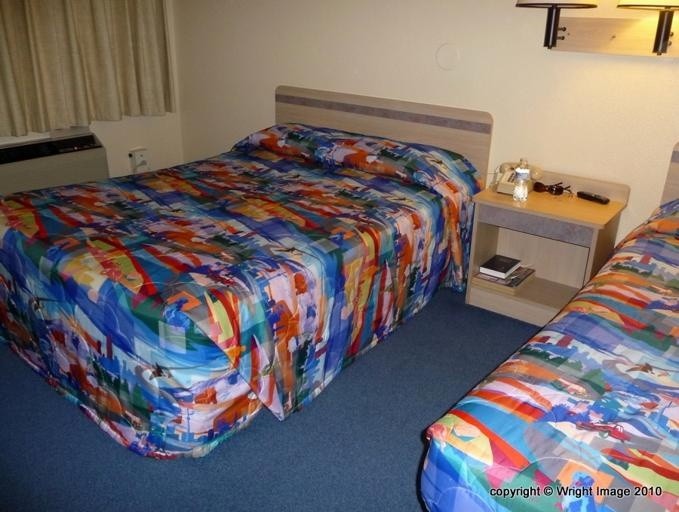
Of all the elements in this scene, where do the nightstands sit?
[463,159,629,329]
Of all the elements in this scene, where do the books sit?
[472,253,536,292]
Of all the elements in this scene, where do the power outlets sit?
[128,147,148,174]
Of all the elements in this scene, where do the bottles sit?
[512,156,528,201]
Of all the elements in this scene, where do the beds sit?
[0,85,493,460]
[415,143,678,511]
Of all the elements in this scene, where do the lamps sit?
[515,1,679,57]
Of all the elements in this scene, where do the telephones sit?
[497,170,535,195]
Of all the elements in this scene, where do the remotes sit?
[576,191,610,205]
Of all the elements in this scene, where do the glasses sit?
[533,181,571,196]
